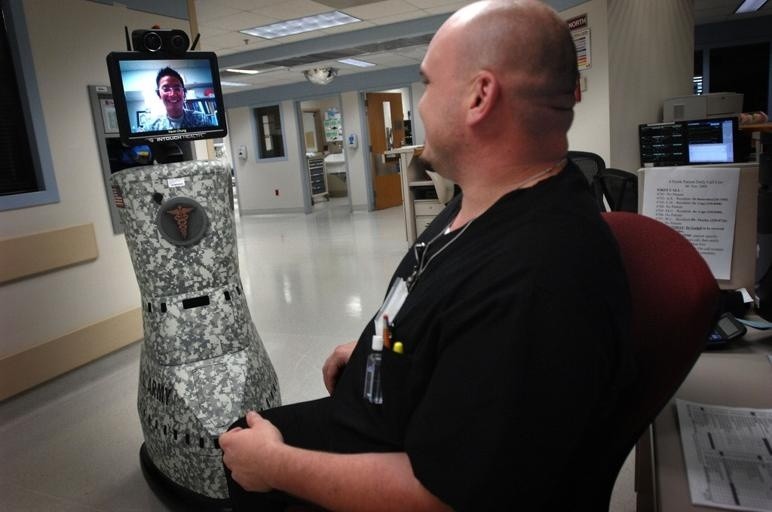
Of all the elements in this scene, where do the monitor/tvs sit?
[106,51,227,146]
[688,116,740,163]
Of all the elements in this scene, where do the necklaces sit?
[409,146,569,292]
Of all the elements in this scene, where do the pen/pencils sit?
[383,315,403,355]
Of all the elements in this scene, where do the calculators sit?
[706,311,746,351]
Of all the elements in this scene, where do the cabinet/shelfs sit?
[385,146,457,248]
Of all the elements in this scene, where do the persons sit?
[138,66,217,132]
[219,2,633,511]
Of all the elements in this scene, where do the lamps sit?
[302,68,339,86]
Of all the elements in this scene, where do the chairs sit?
[599,206,721,512]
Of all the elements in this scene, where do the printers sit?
[662,91,745,122]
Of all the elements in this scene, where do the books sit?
[184,100,216,114]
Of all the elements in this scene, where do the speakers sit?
[131,27,190,52]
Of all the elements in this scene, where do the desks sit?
[635,306,772,512]
